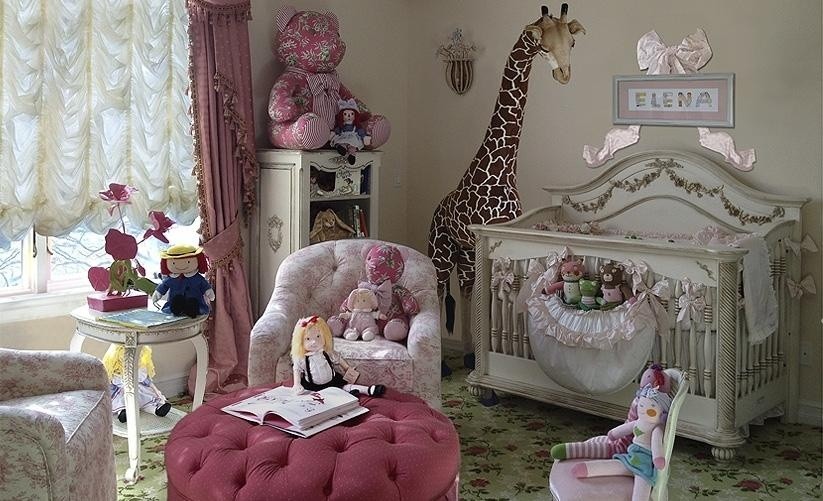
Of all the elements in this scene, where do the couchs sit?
[162,381,459,501]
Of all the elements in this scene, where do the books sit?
[340,203,370,238]
[96,307,188,329]
[220,385,370,440]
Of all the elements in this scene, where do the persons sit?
[152,244,215,321]
[290,316,385,398]
[339,288,389,340]
[330,98,372,165]
[550,366,672,500]
[102,343,172,423]
[310,174,333,199]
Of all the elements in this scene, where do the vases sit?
[81,289,149,312]
[442,56,474,95]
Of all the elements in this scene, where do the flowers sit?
[434,26,479,59]
[83,182,173,297]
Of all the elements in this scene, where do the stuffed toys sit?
[428,4,587,377]
[541,257,637,313]
[310,209,356,244]
[325,245,420,341]
[265,5,391,150]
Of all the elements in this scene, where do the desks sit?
[62,298,212,486]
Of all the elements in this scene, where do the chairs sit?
[245,238,445,418]
[0,344,119,501]
[545,366,691,500]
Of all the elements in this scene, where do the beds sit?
[458,144,813,471]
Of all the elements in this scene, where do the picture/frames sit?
[611,71,736,128]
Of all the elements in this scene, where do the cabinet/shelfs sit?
[233,147,385,339]
